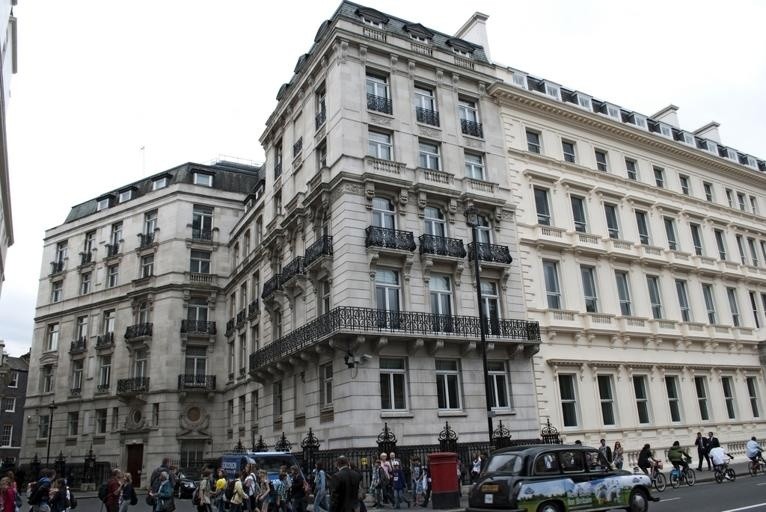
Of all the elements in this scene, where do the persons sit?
[544,439,624,469]
[747,436,765,475]
[694,432,735,482]
[145,457,186,512]
[1,470,75,512]
[372,452,432,509]
[98,468,133,511]
[195,455,368,512]
[638,444,659,483]
[456,450,489,497]
[668,441,691,485]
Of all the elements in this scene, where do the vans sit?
[220,448,310,511]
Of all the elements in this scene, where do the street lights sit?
[463,204,496,456]
[46,400,57,468]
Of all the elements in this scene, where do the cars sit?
[465,443,661,512]
[173,468,202,499]
[200,459,223,503]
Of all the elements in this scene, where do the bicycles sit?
[632,460,667,492]
[670,456,697,489]
[714,457,736,484]
[748,449,766,477]
